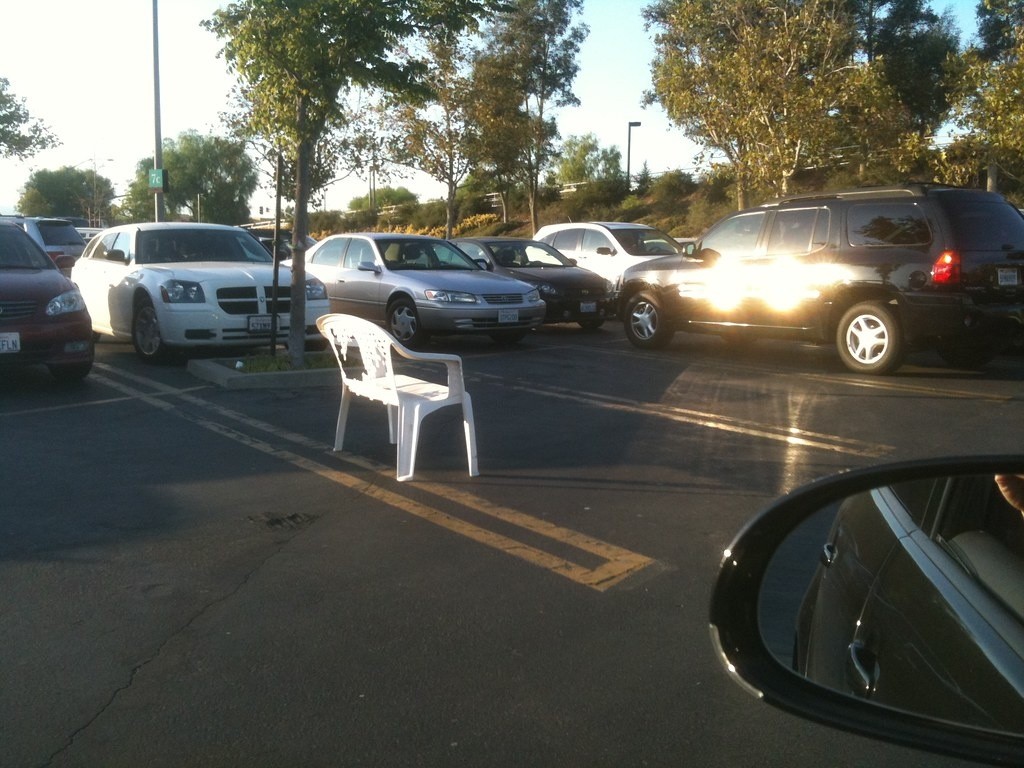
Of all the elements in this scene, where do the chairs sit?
[401,245,427,270]
[501,249,521,267]
[316,313,479,482]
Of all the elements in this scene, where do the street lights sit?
[628,122,643,186]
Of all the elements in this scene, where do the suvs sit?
[1,214,104,277]
[616,179,1024,376]
[525,221,682,284]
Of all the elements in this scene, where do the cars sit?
[444,237,613,331]
[280,231,548,348]
[0,219,95,397]
[70,221,329,363]
[791,471,1021,732]
